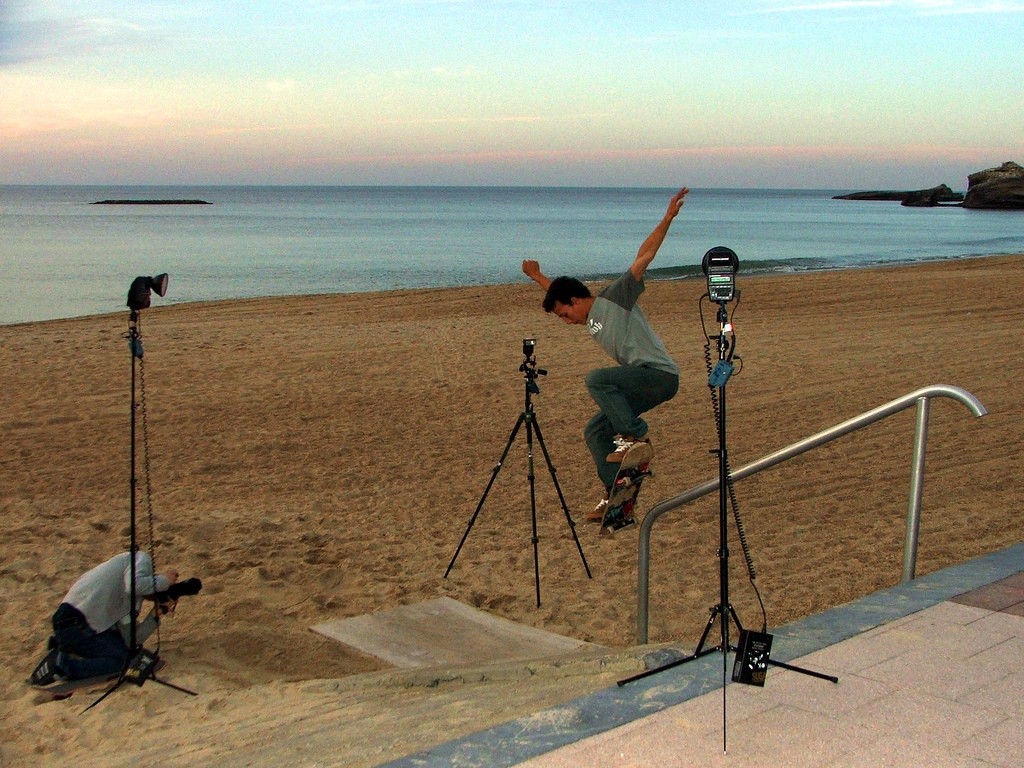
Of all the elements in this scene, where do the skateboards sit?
[595,438,655,539]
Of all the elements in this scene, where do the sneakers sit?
[29,635,59,686]
[606,433,655,462]
[586,491,640,517]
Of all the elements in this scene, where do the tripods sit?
[616,310,838,754]
[78,310,197,715]
[442,356,592,608]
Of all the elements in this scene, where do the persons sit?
[522,187,690,522]
[30,550,180,687]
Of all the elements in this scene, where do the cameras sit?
[523,338,536,356]
[150,577,202,604]
